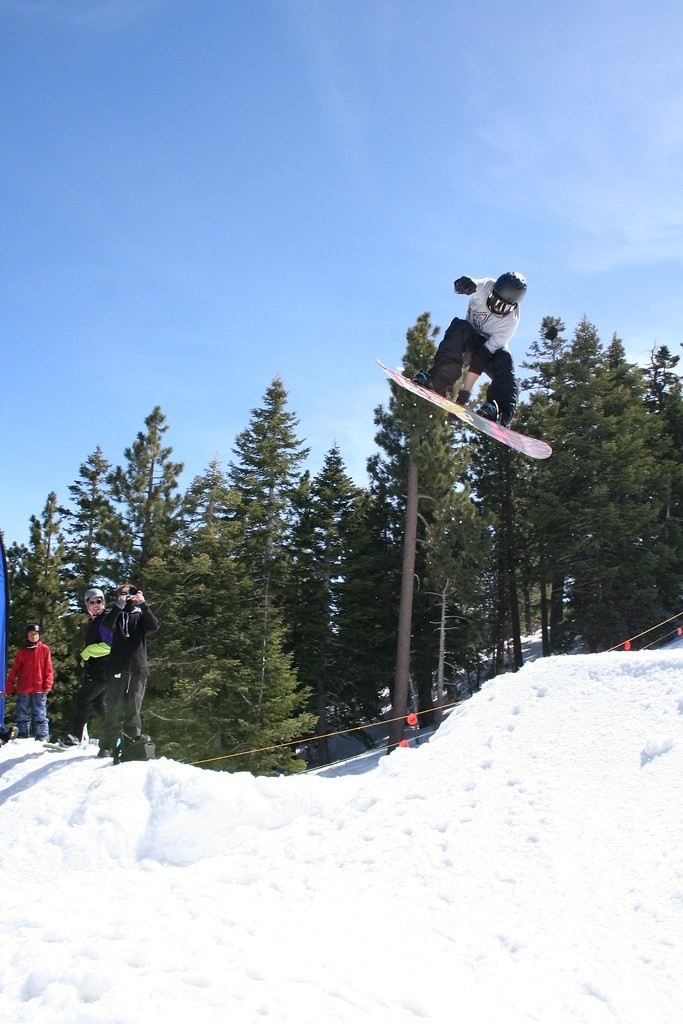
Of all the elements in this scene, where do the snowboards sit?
[42,741,74,750]
[370,356,554,461]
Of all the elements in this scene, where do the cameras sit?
[127,587,138,597]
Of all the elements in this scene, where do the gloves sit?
[456,390,471,408]
[454,276,477,295]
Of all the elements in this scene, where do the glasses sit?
[89,600,103,604]
[118,592,128,595]
[492,292,517,314]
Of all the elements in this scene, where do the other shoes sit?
[98,745,116,757]
[65,735,80,745]
[413,369,453,397]
[477,401,502,423]
[36,735,50,743]
[2,726,19,745]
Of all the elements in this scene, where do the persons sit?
[63,589,120,750]
[97,585,158,757]
[0,691,19,742]
[5,624,54,743]
[413,271,527,426]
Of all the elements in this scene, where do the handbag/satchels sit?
[114,734,155,765]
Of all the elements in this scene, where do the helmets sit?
[487,271,527,317]
[26,624,40,632]
[84,588,104,602]
